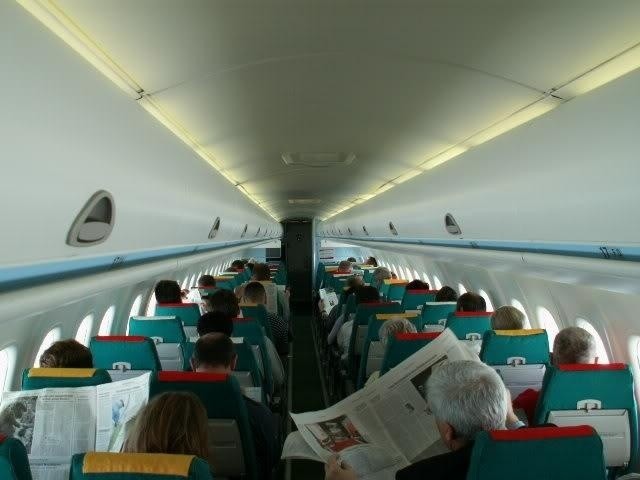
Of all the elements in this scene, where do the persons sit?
[189,333,276,478]
[325,360,557,479]
[119,390,212,462]
[154,257,291,356]
[40,338,94,369]
[548,328,599,365]
[319,256,525,389]
